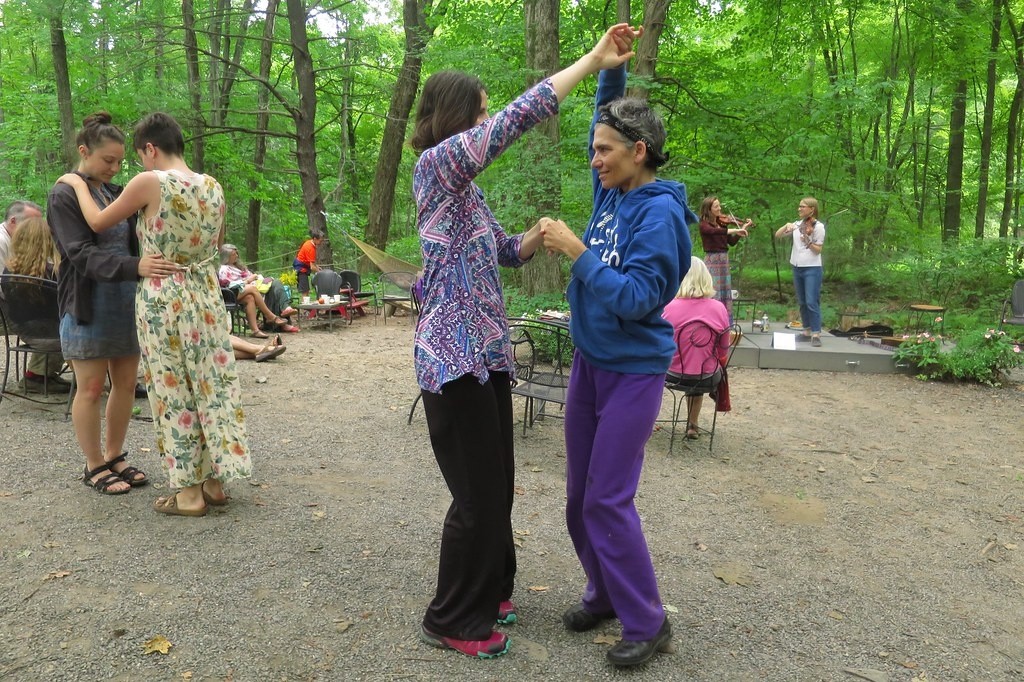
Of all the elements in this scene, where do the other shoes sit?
[811,337,822,347]
[795,334,811,342]
[135,383,147,398]
[281,309,298,317]
[283,326,300,332]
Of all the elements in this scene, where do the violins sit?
[798,216,816,249]
[716,213,757,228]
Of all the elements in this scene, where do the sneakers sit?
[497,601,517,624]
[18,373,71,394]
[420,622,510,660]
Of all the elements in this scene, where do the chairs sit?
[220,286,300,336]
[377,272,415,326]
[729,260,758,333]
[999,280,1024,349]
[657,319,742,451]
[409,285,534,435]
[904,269,954,343]
[507,317,575,437]
[311,270,380,325]
[0,273,112,422]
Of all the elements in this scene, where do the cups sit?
[303,297,310,304]
[731,289,738,300]
[321,295,328,300]
[324,296,330,304]
[334,295,340,303]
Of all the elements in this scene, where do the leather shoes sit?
[563,602,617,632]
[607,616,671,665]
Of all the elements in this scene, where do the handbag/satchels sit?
[256,277,274,294]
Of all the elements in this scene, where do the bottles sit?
[761,313,769,332]
[329,297,335,304]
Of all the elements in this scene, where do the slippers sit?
[153,491,209,516]
[201,480,227,506]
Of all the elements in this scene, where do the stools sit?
[836,312,866,330]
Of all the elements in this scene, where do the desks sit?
[538,319,569,410]
[296,301,349,333]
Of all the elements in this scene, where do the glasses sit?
[798,206,812,209]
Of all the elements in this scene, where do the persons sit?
[699,196,752,332]
[543,26,697,669]
[775,197,825,348]
[46,111,178,496]
[56,113,251,516]
[293,230,325,316]
[414,24,635,658]
[218,244,301,363]
[0,200,153,399]
[662,257,730,441]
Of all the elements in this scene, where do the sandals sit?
[267,316,288,326]
[84,465,131,495]
[249,330,269,339]
[271,334,283,346]
[687,425,698,439]
[105,452,148,485]
[255,345,286,362]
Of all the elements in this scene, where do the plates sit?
[788,324,806,330]
